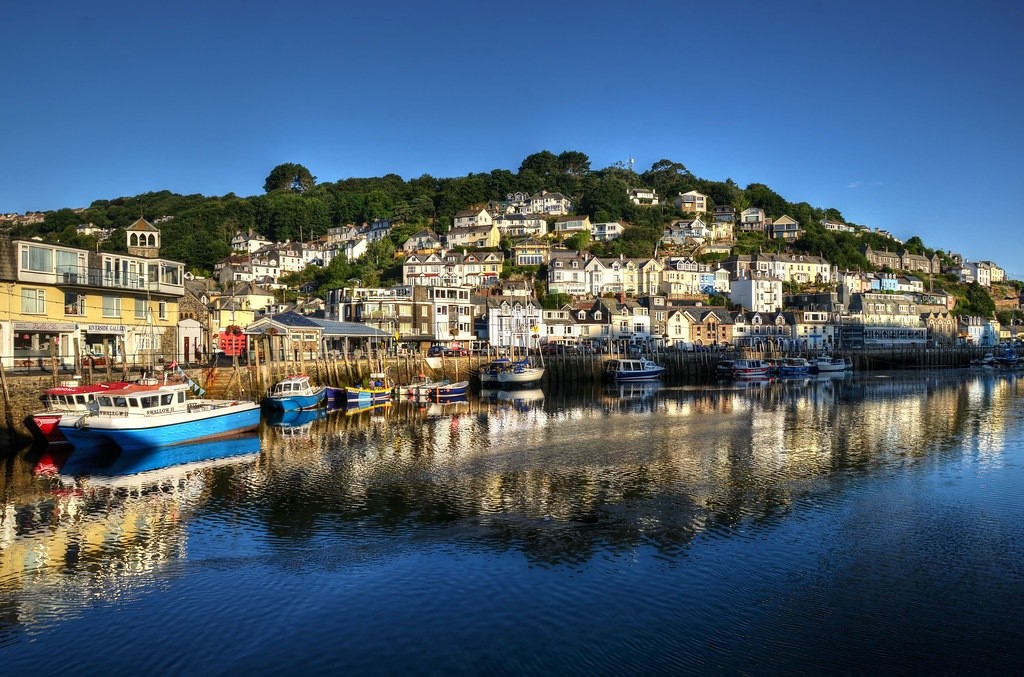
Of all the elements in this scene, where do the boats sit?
[477,356,546,382]
[816,352,846,371]
[327,371,470,402]
[995,348,1019,363]
[262,375,327,411]
[57,381,262,451]
[31,380,132,447]
[605,359,666,381]
[716,358,770,374]
[764,357,810,374]
